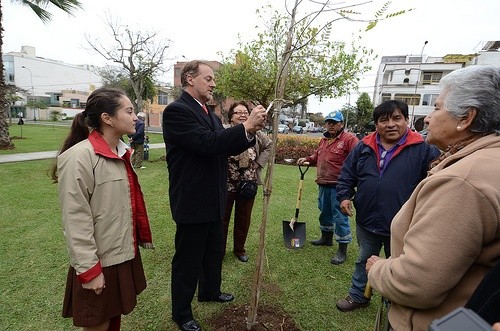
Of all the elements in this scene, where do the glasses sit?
[233,111,249,116]
[379,151,388,170]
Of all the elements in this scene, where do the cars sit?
[306,126,326,133]
[269,124,290,135]
[292,126,303,134]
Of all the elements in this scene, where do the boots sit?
[331,244,348,265]
[311,230,334,246]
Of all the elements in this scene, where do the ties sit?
[202,105,208,114]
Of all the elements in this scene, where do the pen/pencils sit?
[250,100,257,107]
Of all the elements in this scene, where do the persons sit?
[336,100,441,311]
[366,65,500,331]
[162,61,267,331]
[127,112,146,168]
[297,111,360,265]
[224,100,272,262]
[48,88,154,331]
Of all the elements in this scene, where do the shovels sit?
[283,162,311,250]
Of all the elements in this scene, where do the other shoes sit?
[336,295,371,312]
[138,166,146,169]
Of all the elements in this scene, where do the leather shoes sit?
[197,292,234,303]
[172,319,202,331]
[234,251,248,263]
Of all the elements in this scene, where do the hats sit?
[324,111,343,121]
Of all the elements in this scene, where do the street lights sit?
[410,40,429,128]
[21,66,35,121]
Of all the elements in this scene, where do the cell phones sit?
[427,307,494,331]
